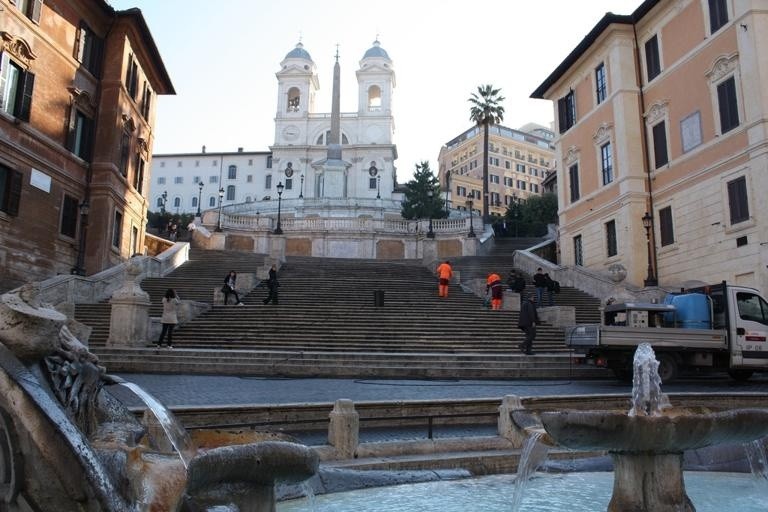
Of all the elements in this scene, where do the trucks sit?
[564,279,768,384]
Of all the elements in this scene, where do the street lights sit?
[215,187,225,231]
[71,200,90,276]
[162,190,168,209]
[299,174,304,198]
[512,191,519,241]
[196,182,204,216]
[641,213,657,286]
[376,174,381,199]
[273,182,284,235]
[426,184,490,238]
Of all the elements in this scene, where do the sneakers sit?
[157,345,173,349]
[238,302,244,306]
[519,344,536,355]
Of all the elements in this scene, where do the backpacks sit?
[554,281,560,294]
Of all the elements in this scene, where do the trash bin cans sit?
[375,291,384,306]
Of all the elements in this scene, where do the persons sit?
[506,269,526,303]
[149,212,195,242]
[544,273,560,306]
[436,260,453,298]
[263,264,280,305]
[221,270,244,306]
[518,292,540,355]
[534,267,545,307]
[157,288,181,349]
[485,270,503,311]
